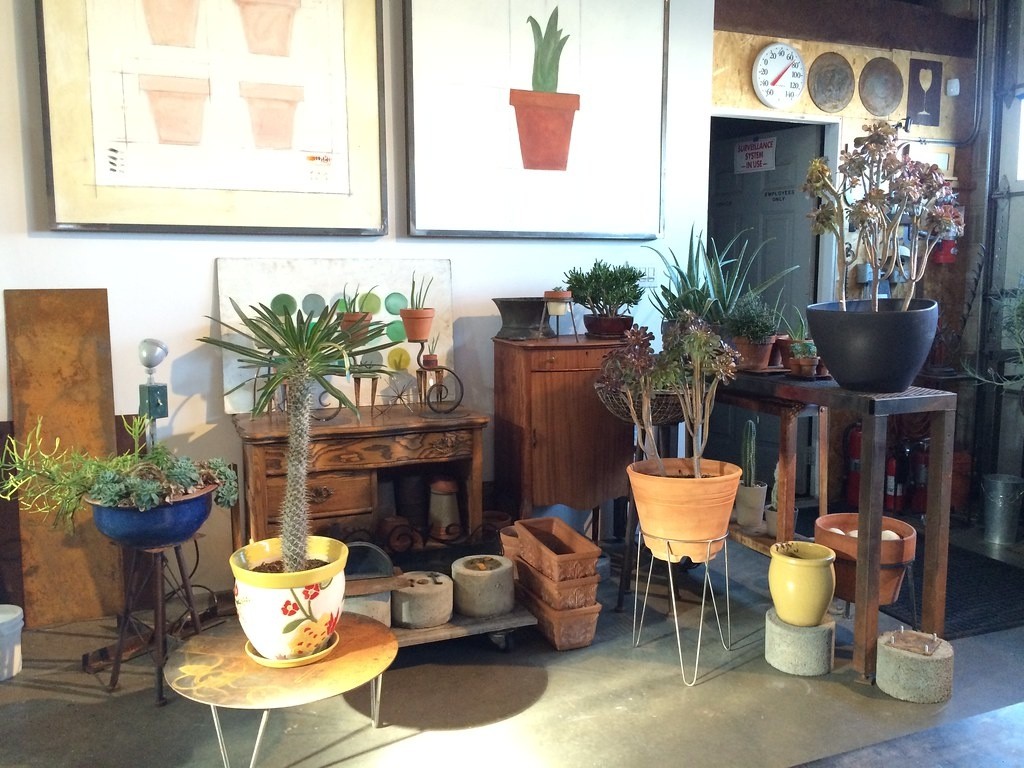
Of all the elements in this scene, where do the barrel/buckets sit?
[981,474,1024,546]
[812,511,918,607]
[0,604,24,682]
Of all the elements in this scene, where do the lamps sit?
[139,339,169,449]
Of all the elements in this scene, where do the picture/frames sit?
[402,0,672,243]
[34,0,389,237]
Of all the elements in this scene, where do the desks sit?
[686,371,957,687]
[163,613,398,768]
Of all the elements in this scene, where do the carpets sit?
[796,503,1024,639]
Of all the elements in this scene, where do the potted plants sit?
[547,116,978,564]
[1,272,439,668]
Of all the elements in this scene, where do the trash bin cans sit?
[981,472,1024,546]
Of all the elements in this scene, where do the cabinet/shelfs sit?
[489,336,637,539]
[232,400,490,567]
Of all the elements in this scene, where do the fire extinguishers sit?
[885,438,914,519]
[843,416,862,507]
[909,437,931,514]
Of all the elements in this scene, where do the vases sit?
[766,539,835,627]
[486,510,601,651]
[815,513,917,606]
